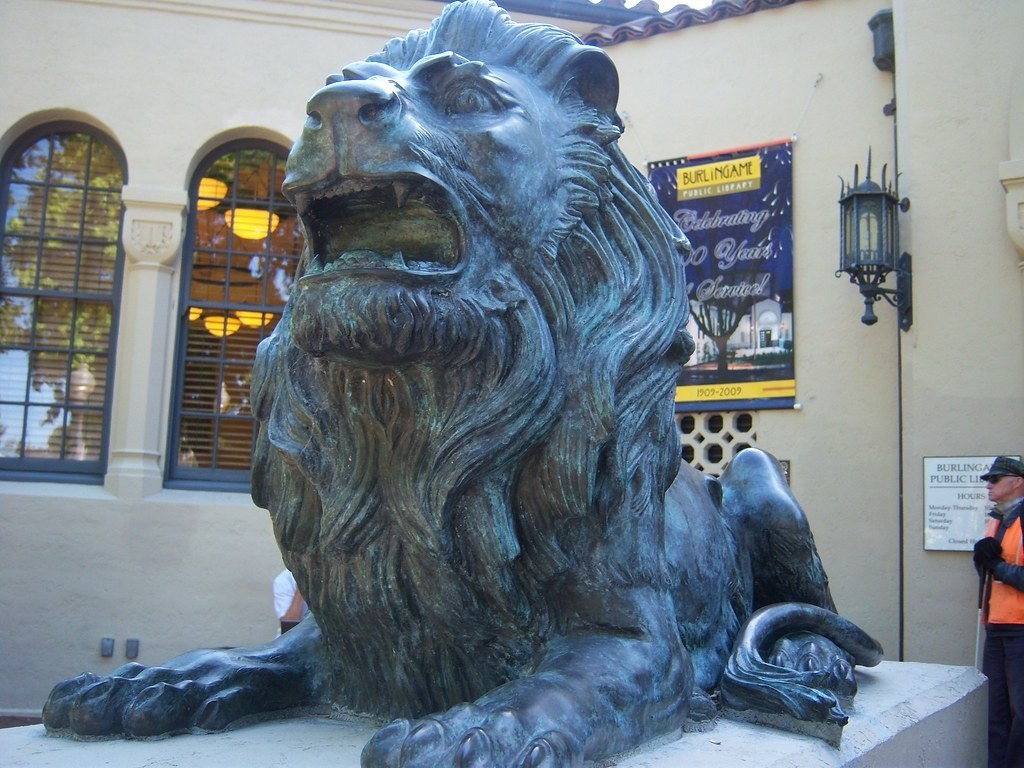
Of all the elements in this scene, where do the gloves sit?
[974,537,1005,575]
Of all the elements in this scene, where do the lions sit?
[41,1,886,767]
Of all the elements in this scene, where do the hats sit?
[980,455,1024,480]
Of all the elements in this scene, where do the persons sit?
[273,570,308,638]
[973,456,1023,768]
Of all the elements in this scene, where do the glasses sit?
[988,474,1019,484]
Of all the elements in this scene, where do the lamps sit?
[198,168,294,240]
[835,145,913,334]
[188,307,274,337]
